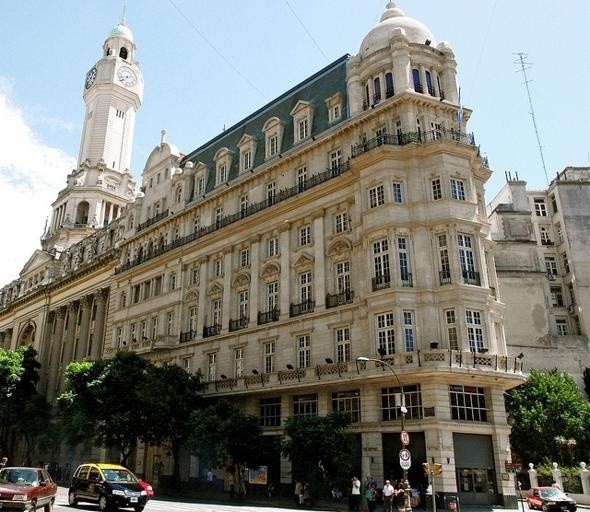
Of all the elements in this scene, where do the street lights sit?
[356,356,413,512]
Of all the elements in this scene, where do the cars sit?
[0,462,154,512]
[525,486,578,512]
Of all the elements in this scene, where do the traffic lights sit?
[421,463,442,475]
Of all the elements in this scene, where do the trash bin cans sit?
[425,493,433,512]
[444,494,461,512]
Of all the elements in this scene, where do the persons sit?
[227,471,238,499]
[552,480,561,490]
[16,461,135,486]
[239,469,247,495]
[205,469,214,490]
[0,456,8,470]
[292,473,440,512]
[258,471,265,481]
[268,483,275,498]
